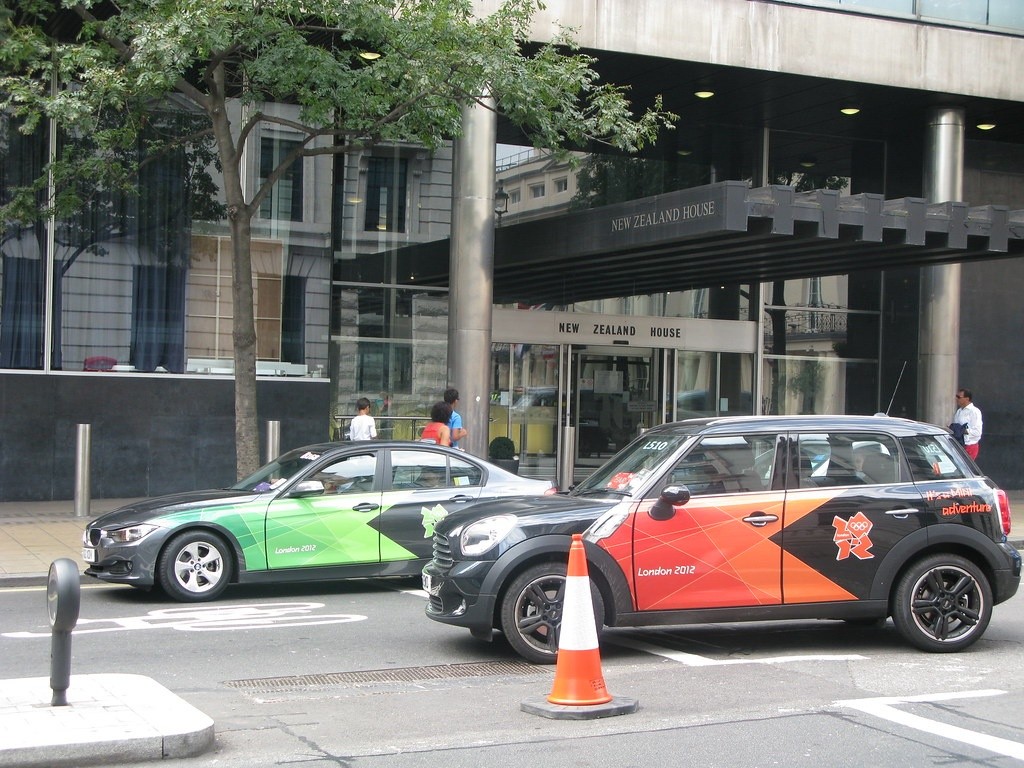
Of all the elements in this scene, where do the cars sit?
[423,413,1023,667]
[669,387,754,413]
[81,439,561,604]
[515,390,559,408]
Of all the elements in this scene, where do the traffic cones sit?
[521,532,640,722]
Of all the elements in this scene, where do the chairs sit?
[861,453,893,483]
[737,445,762,489]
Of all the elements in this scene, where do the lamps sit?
[360,52,382,60]
[692,85,716,99]
[839,104,861,116]
[974,118,998,131]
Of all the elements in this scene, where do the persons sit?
[699,450,735,493]
[325,462,367,494]
[949,388,983,460]
[349,398,377,442]
[422,469,440,488]
[853,448,865,472]
[420,388,467,446]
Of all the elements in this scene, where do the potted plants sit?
[489,437,520,475]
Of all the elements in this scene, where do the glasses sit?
[456,397,460,401]
[955,394,964,399]
